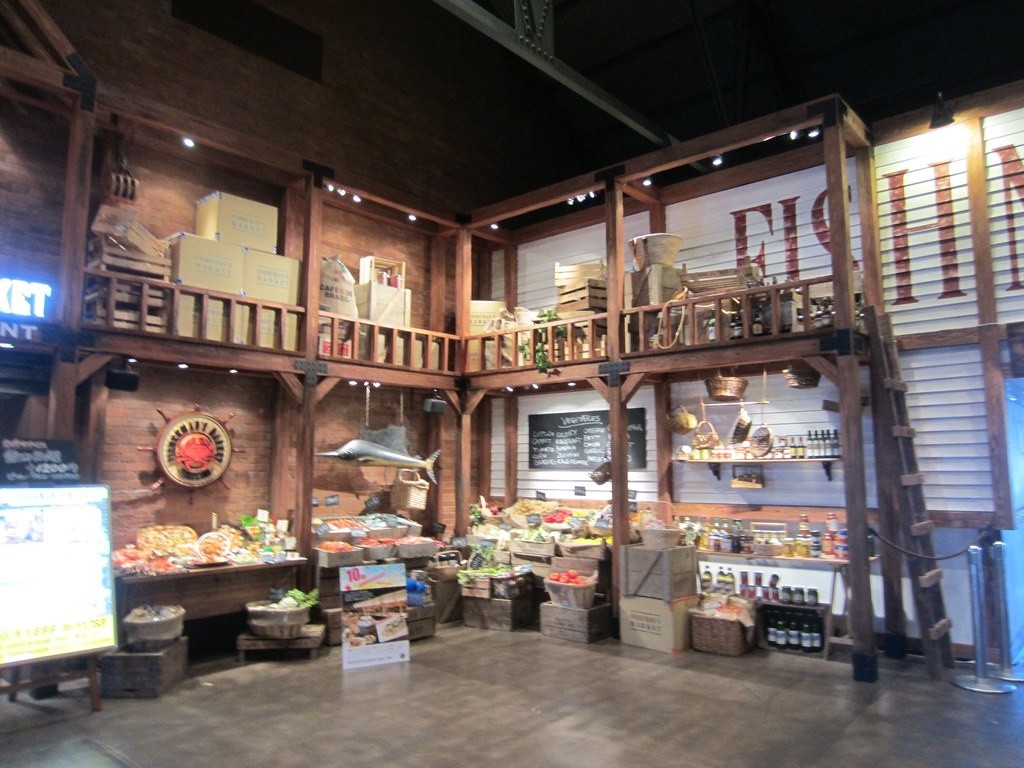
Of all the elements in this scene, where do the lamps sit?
[928,92,956,130]
[105,364,141,392]
[807,126,821,138]
[712,155,723,166]
[424,390,448,414]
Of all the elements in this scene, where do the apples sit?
[550,568,593,585]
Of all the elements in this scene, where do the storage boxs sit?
[314,511,702,672]
[101,634,189,702]
[162,190,688,375]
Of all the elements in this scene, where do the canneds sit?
[738,571,818,606]
[708,534,751,554]
[784,513,850,560]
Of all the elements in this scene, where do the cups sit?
[262,551,301,561]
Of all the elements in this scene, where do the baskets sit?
[664,405,697,435]
[750,423,773,457]
[627,232,684,273]
[434,550,467,581]
[587,456,612,485]
[691,606,747,657]
[636,501,681,550]
[782,359,821,389]
[705,366,748,401]
[389,468,430,511]
[691,421,719,449]
[729,408,752,444]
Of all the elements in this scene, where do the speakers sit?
[424,399,447,414]
[106,369,140,391]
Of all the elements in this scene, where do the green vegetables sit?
[286,587,319,610]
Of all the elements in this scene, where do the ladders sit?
[863,305,954,681]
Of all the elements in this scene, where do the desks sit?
[114,551,307,647]
[701,548,881,661]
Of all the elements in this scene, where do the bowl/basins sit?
[676,452,690,460]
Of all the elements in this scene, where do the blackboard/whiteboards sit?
[0,437,81,488]
[529,408,646,472]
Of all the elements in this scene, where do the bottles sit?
[765,609,823,654]
[357,616,375,634]
[691,428,841,461]
[210,512,220,533]
[440,553,458,567]
[631,505,658,527]
[728,275,782,344]
[708,308,716,343]
[375,266,402,289]
[868,535,875,557]
[673,511,849,560]
[595,500,613,530]
[518,307,567,368]
[783,275,867,334]
[701,565,819,606]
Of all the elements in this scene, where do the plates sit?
[285,557,308,561]
[345,635,376,648]
[187,532,260,566]
